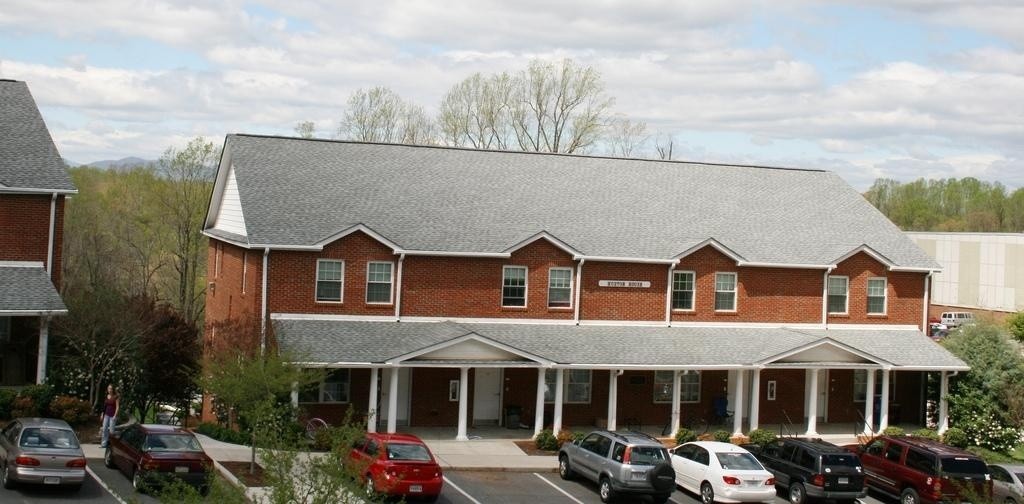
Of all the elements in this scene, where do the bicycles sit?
[296,412,329,440]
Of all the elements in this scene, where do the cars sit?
[100,421,213,493]
[984,463,1024,503]
[329,432,443,500]
[930,322,953,343]
[0,416,86,493]
[662,439,777,504]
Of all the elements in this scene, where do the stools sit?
[624,417,642,432]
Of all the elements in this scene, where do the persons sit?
[99,384,120,448]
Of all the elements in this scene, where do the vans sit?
[940,311,974,328]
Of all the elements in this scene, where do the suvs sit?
[841,432,993,504]
[738,436,867,503]
[557,427,676,504]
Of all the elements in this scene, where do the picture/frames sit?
[449,380,459,402]
[767,381,776,400]
